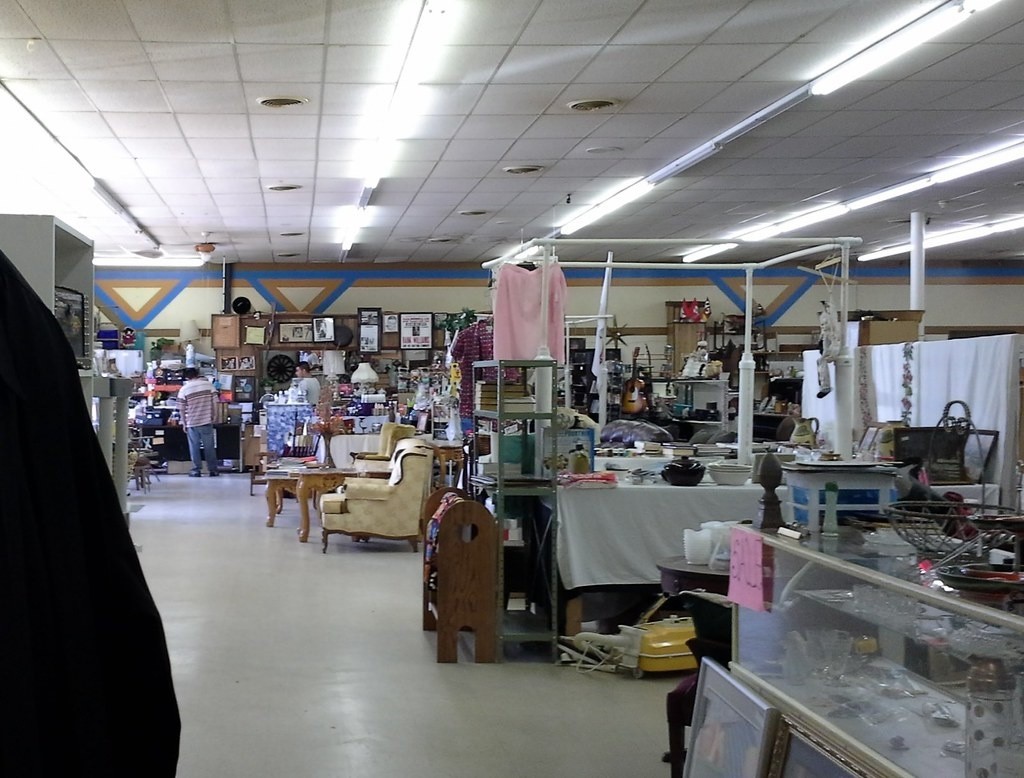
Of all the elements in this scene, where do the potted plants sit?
[148,337,175,360]
[259,377,278,394]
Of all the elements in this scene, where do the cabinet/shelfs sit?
[422,295,1024,778]
[0,214,92,425]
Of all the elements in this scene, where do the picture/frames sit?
[209,307,448,404]
[54,285,91,370]
[679,655,779,778]
[859,424,880,451]
[766,715,875,778]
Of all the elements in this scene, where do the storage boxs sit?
[398,392,415,405]
[817,309,925,347]
[673,403,693,417]
[508,527,523,541]
[506,598,527,611]
[540,427,594,478]
[653,395,678,405]
[706,401,718,410]
[368,394,386,403]
[632,617,699,677]
[140,360,201,424]
[694,408,721,421]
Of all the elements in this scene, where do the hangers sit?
[513,245,543,268]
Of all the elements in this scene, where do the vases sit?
[323,432,336,467]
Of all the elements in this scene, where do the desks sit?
[556,474,765,646]
[263,467,357,544]
[315,434,433,468]
[141,424,243,473]
[930,483,1001,516]
[429,438,464,490]
[657,555,730,778]
[93,375,134,530]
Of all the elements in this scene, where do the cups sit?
[784,623,854,687]
[962,674,1016,778]
[850,583,921,635]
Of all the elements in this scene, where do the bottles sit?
[374,402,385,416]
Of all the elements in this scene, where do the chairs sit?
[128,427,161,495]
[314,421,435,553]
[250,453,278,496]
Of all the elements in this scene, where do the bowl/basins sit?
[660,455,706,486]
[705,463,753,485]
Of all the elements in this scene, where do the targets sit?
[266,354,297,384]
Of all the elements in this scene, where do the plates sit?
[877,527,941,543]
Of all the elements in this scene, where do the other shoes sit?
[189,470,201,476]
[210,471,219,476]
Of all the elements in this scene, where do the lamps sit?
[350,362,380,394]
[0,0,1024,273]
[323,349,346,402]
[179,319,201,368]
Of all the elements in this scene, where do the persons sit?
[295,362,320,410]
[178,368,219,477]
[0,251,181,778]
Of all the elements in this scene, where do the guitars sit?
[621,346,650,415]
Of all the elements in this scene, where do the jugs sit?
[789,417,821,449]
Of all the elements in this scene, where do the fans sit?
[163,231,244,254]
